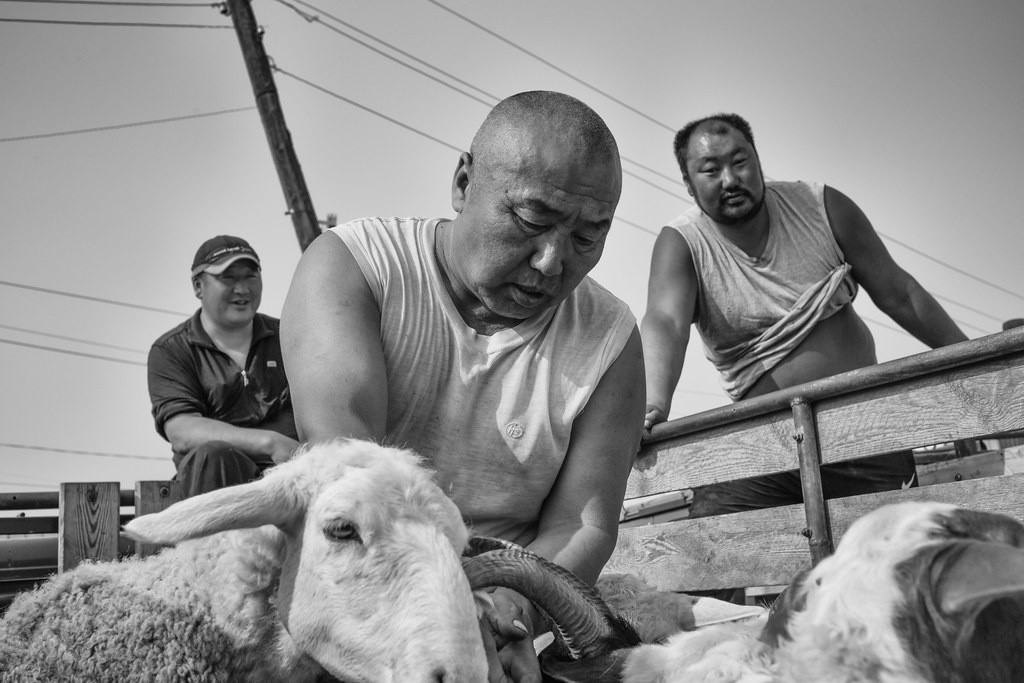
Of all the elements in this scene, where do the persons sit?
[147,234,302,504]
[637,114,971,606]
[277,92,646,681]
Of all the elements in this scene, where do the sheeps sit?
[0,435,490,683]
[465,499,1024,683]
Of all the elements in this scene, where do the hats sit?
[190,235,262,279]
